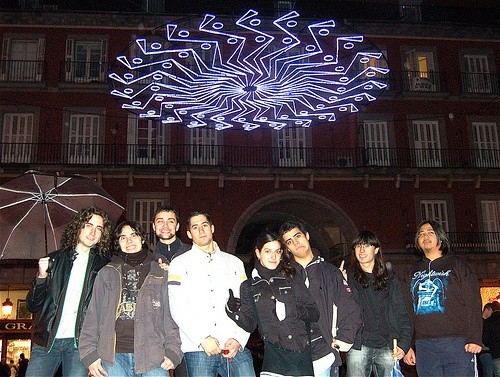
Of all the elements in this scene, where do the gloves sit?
[225,289,241,313]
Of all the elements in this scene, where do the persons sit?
[336,229,412,377]
[152,206,190,377]
[23,206,114,377]
[0,352,30,377]
[402,218,484,377]
[279,218,363,377]
[78,220,184,377]
[477,300,500,377]
[167,209,257,377]
[225,231,320,377]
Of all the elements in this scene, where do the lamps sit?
[2,285,13,318]
[105,8,392,130]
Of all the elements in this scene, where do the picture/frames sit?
[16,299,33,321]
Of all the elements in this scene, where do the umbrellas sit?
[0,168,129,272]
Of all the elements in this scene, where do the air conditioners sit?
[337,156,353,167]
